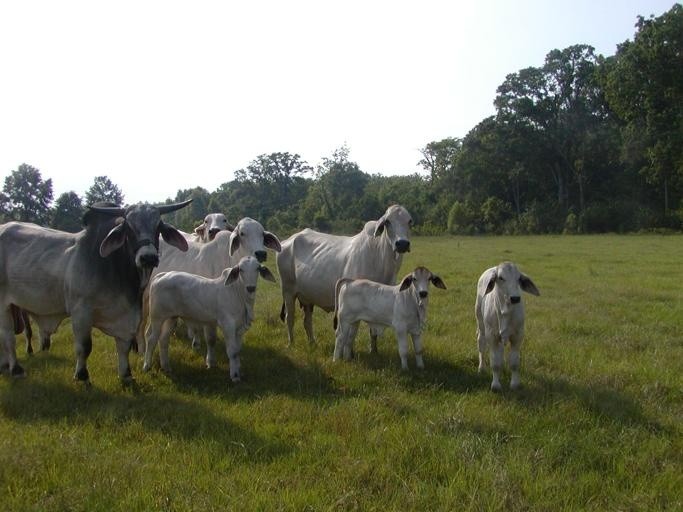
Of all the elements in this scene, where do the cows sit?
[276,202,414,356]
[332,267,448,374]
[0,198,194,396]
[473,260,540,395]
[135,212,282,385]
